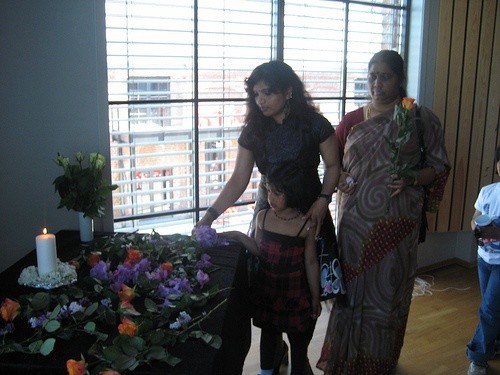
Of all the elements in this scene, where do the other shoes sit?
[468,362,489,375]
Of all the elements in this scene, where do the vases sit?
[77,212,93,242]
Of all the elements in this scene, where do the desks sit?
[0,229,252,375]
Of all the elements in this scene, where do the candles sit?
[35,228,57,277]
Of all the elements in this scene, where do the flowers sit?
[0,228,231,375]
[52,150,118,220]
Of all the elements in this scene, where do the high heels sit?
[281,340,289,366]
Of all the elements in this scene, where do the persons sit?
[317,51,451,374]
[194,61,342,375]
[216,161,322,375]
[466,147,500,375]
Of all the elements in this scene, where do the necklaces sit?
[365,105,371,121]
[273,212,299,220]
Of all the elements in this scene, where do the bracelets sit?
[319,194,332,202]
[208,208,218,219]
